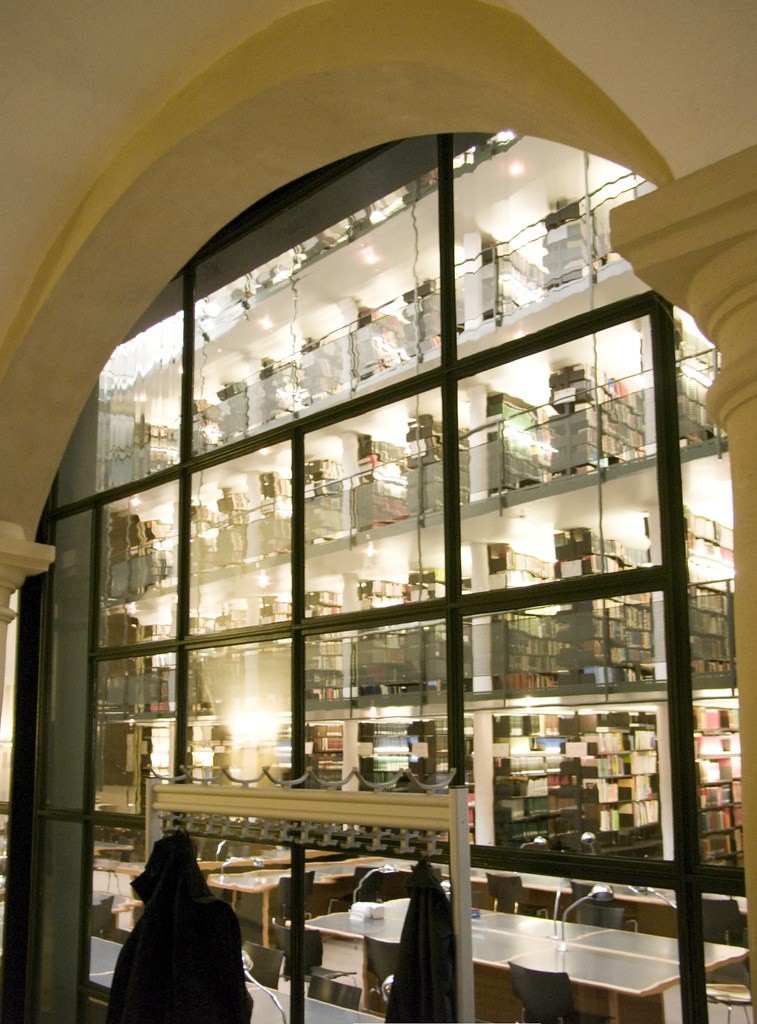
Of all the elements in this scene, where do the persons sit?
[106,831,253,1024]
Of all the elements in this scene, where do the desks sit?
[91,936,387,1024]
[91,841,392,929]
[285,897,750,1024]
[470,868,748,946]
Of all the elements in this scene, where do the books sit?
[81,118,744,908]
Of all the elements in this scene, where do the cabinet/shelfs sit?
[97,140,746,870]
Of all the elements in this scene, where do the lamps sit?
[219,856,265,882]
[520,836,547,848]
[557,885,613,952]
[581,831,597,856]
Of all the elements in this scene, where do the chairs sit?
[94,842,751,1024]
